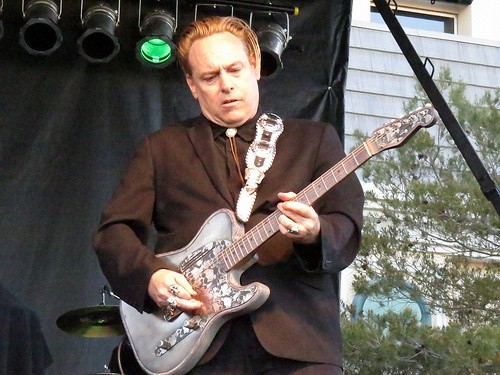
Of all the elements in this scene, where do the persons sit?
[92,16,367,375]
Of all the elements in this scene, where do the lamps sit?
[136,0,178,69]
[249,11,292,77]
[17,0,64,56]
[77,0,121,63]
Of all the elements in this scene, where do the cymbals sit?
[56,306,129,336]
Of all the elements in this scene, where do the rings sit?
[287,226,299,234]
[166,297,178,309]
[166,306,174,316]
[169,285,181,296]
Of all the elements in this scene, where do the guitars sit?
[119,105,438,375]
